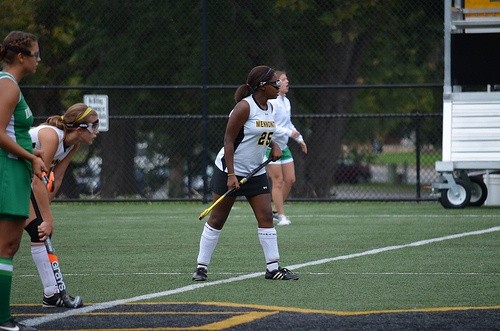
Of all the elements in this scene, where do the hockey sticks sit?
[198,155,274,220]
[37,155,56,192]
[29,188,84,309]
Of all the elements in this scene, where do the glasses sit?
[87,119,99,134]
[32,51,40,60]
[270,79,281,89]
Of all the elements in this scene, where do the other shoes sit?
[273,211,279,220]
[278,215,291,225]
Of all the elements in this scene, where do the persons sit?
[0,30,49,331]
[191,66,301,281]
[267,70,307,225]
[24,103,100,308]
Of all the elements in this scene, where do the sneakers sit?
[192,267,207,280]
[0,317,37,331]
[265,266,299,280]
[43,292,83,308]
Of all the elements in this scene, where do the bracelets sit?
[227,173,234,176]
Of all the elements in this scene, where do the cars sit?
[59,129,173,199]
